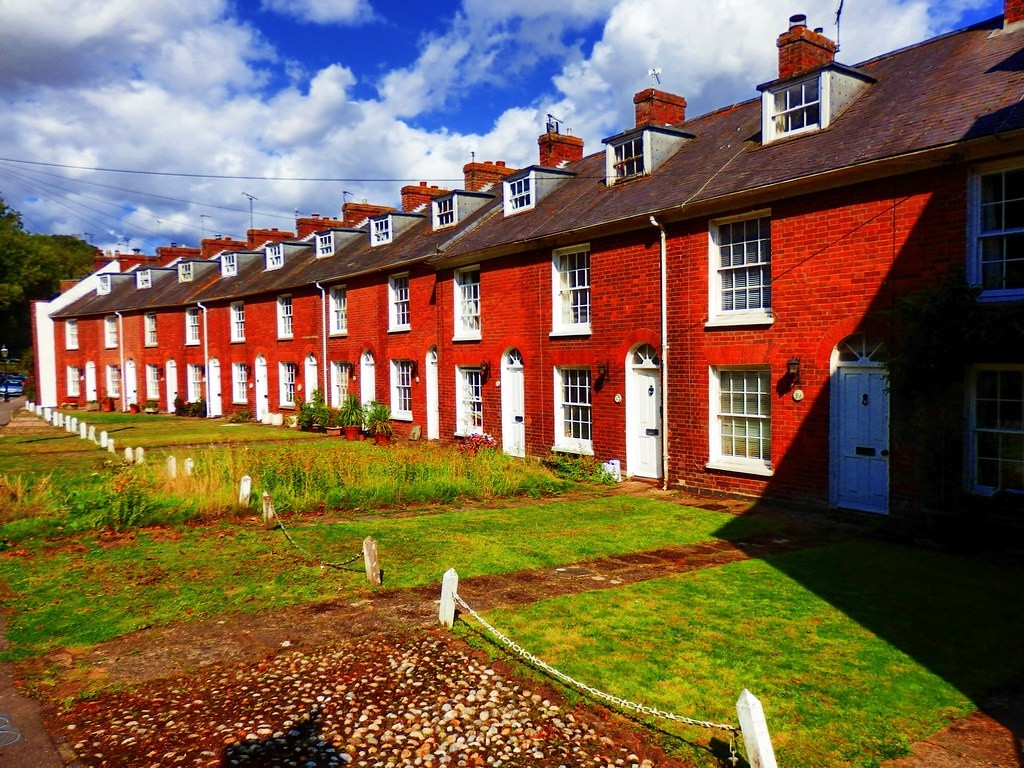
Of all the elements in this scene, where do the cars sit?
[0,372,29,386]
[0,379,25,396]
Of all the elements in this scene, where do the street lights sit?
[1,345,9,403]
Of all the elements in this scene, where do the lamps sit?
[598,358,609,383]
[244,363,252,374]
[347,361,355,373]
[408,359,418,375]
[292,361,300,372]
[786,357,800,385]
[480,360,491,378]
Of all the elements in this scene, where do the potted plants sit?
[61,401,78,410]
[86,396,114,412]
[173,395,205,418]
[283,388,394,447]
[129,400,159,414]
